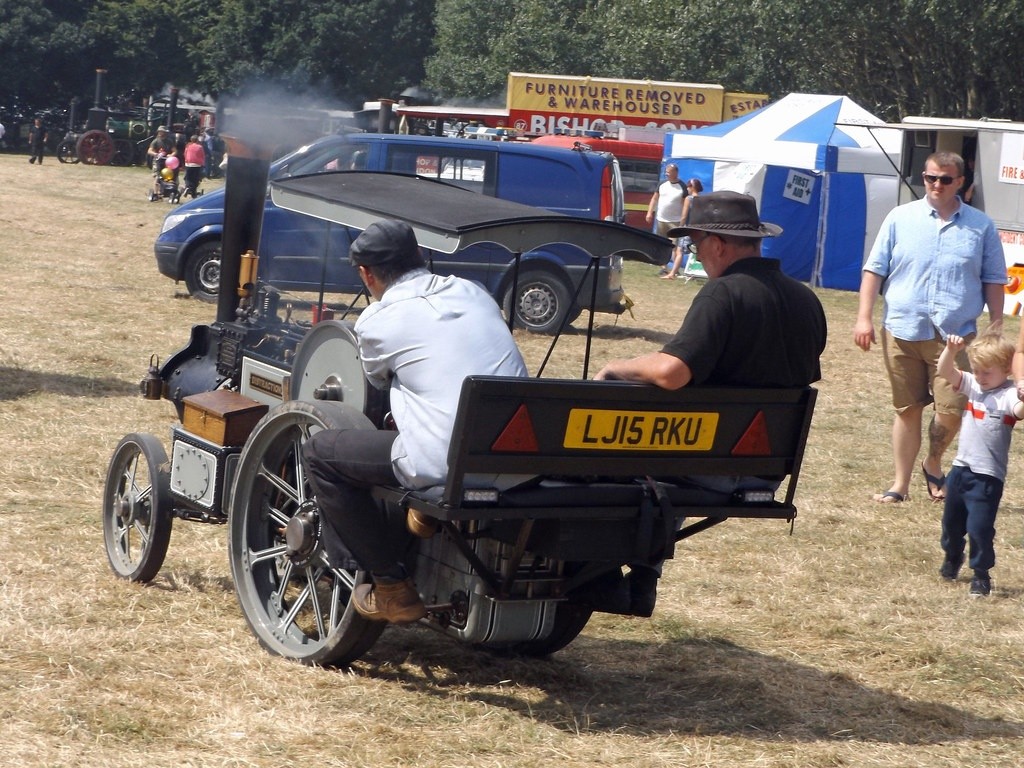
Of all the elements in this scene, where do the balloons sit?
[161,156,180,179]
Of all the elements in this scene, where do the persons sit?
[184,134,206,199]
[592,190,828,618]
[198,128,224,178]
[663,179,703,280]
[148,125,178,194]
[646,163,689,276]
[301,219,530,623]
[29,118,48,165]
[854,152,1007,504]
[937,320,1024,597]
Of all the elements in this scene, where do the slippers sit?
[921,461,947,500]
[878,491,911,503]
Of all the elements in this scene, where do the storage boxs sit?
[182,389,269,447]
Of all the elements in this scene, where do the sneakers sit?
[408,508,438,536]
[352,584,426,622]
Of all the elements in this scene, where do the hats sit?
[157,126,170,132]
[667,191,783,237]
[350,219,417,267]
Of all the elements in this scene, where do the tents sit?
[660,93,902,292]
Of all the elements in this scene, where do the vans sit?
[150,134,626,337]
[528,136,665,241]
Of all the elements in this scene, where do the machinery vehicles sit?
[101,134,822,671]
[55,67,200,166]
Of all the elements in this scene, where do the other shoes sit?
[659,275,674,280]
[969,574,990,597]
[939,552,965,582]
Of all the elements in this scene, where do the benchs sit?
[369,376,818,521]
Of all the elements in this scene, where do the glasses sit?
[923,175,961,185]
[686,235,727,255]
